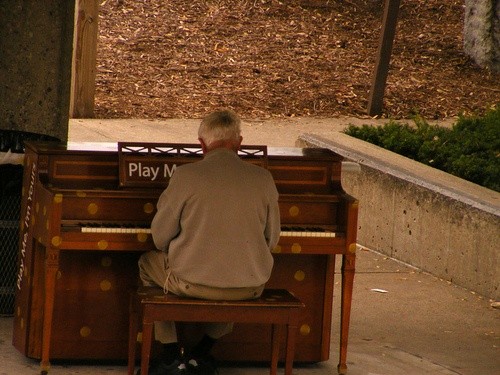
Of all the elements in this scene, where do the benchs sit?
[127,288,308,375]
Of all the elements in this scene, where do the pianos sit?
[12,142,358,375]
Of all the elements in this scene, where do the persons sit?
[138,111,280,375]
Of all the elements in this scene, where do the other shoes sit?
[136,358,215,375]
